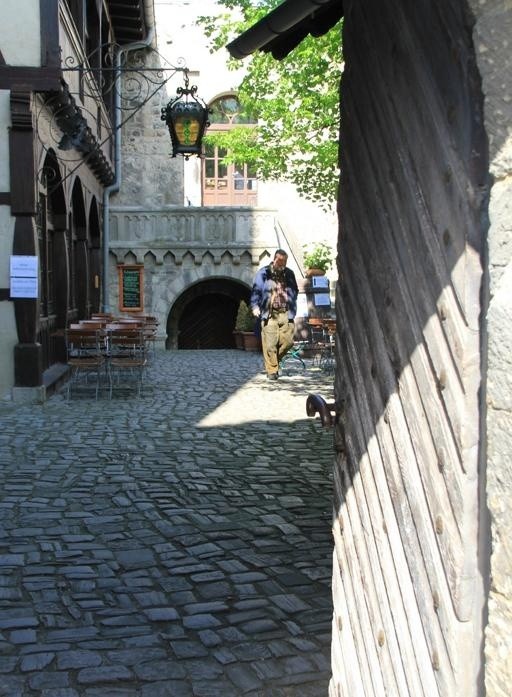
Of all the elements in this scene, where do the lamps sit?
[32,39,213,205]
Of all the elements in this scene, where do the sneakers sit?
[268,373,278,380]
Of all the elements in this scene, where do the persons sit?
[250,250,299,379]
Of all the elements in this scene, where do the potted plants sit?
[241,305,262,352]
[232,300,249,349]
[302,241,333,277]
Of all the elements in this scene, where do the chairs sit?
[282,319,336,377]
[64,313,160,401]
